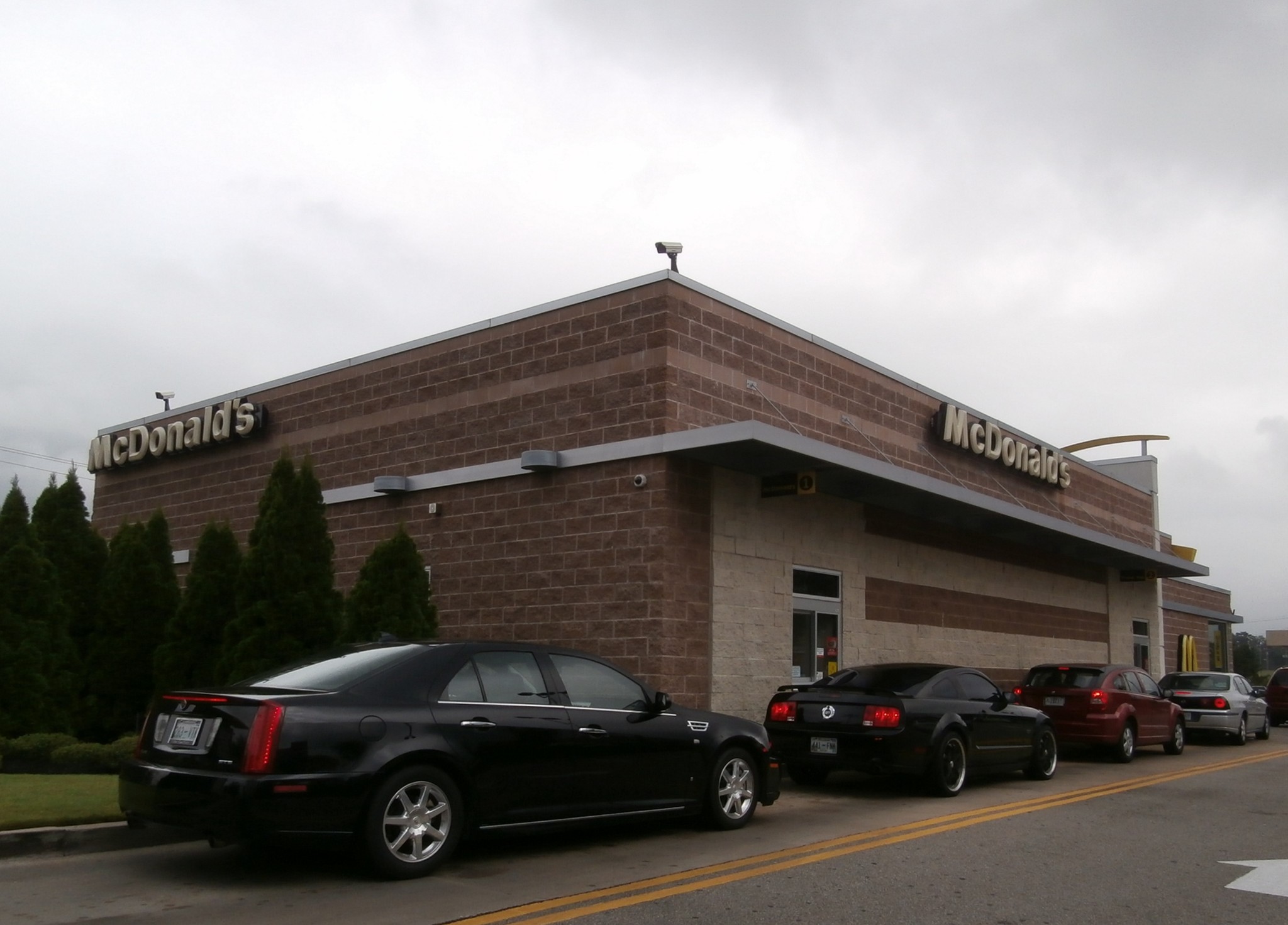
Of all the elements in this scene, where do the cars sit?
[1157,671,1270,746]
[118,640,781,879]
[764,662,1063,800]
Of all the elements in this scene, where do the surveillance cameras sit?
[655,242,683,254]
[633,474,646,487]
[155,392,174,400]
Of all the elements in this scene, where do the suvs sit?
[1267,667,1288,727]
[1013,663,1186,763]
